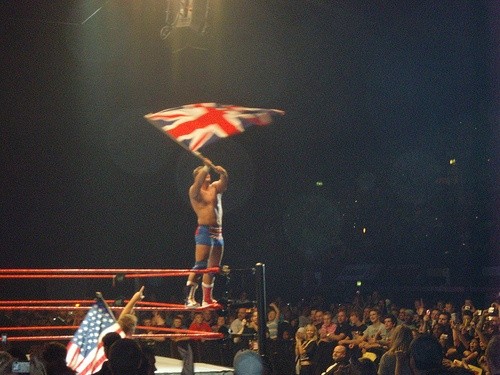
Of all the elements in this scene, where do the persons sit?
[118,285,146,336]
[186,312,212,362]
[64,292,500,375]
[0,332,157,375]
[184,158,229,307]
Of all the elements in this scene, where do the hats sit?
[234,350,264,375]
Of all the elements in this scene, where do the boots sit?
[201,283,218,307]
[184,279,200,308]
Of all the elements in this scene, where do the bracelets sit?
[326,333,331,337]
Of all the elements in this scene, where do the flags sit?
[65,299,126,375]
[147,102,286,152]
